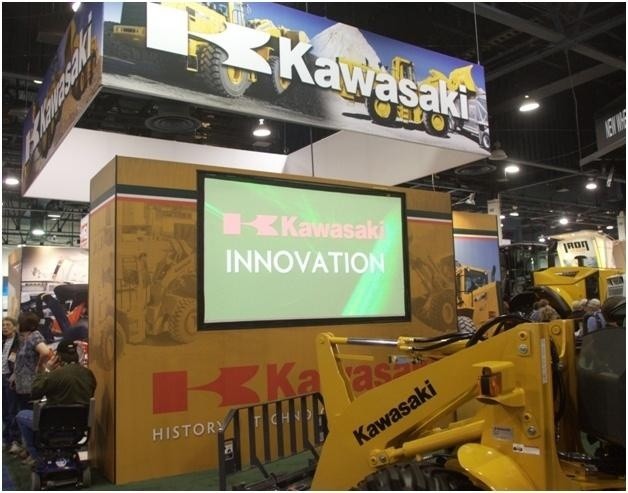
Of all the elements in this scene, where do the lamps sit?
[519,95,539,112]
[586,178,598,190]
[32,223,44,235]
[253,119,271,136]
[464,192,476,205]
[488,141,508,161]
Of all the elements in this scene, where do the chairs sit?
[27,398,95,491]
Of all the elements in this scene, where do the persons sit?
[9,308,51,456]
[15,339,98,465]
[531,298,607,341]
[3,317,24,455]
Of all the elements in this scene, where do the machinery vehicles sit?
[113,2,310,98]
[54,18,98,101]
[456,259,502,339]
[218,315,626,491]
[335,55,478,136]
[499,239,626,319]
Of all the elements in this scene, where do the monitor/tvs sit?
[195,169,412,332]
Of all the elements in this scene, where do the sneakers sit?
[3,442,35,466]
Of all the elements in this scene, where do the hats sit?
[55,341,76,350]
